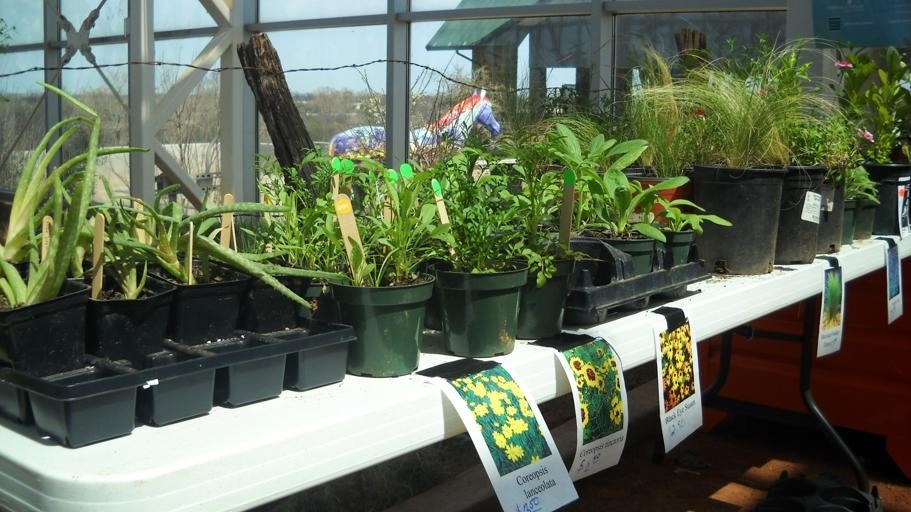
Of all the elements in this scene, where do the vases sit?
[691,157,911,277]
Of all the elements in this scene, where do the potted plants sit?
[0,29,910,372]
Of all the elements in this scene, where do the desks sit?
[0,230,911,512]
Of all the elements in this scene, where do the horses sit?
[328,86,501,161]
